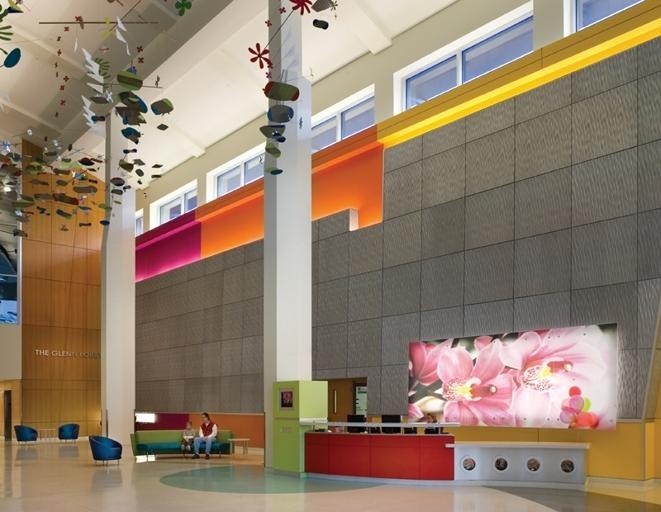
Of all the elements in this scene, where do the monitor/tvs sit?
[382,414,401,433]
[347,414,367,433]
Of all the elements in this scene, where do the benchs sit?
[130,430,233,462]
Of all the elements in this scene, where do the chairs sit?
[59,424,79,443]
[14,425,38,445]
[89,435,122,466]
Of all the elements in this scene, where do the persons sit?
[423,413,444,434]
[180,420,196,451]
[192,411,218,460]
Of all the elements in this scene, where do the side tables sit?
[39,429,56,441]
[228,438,250,456]
